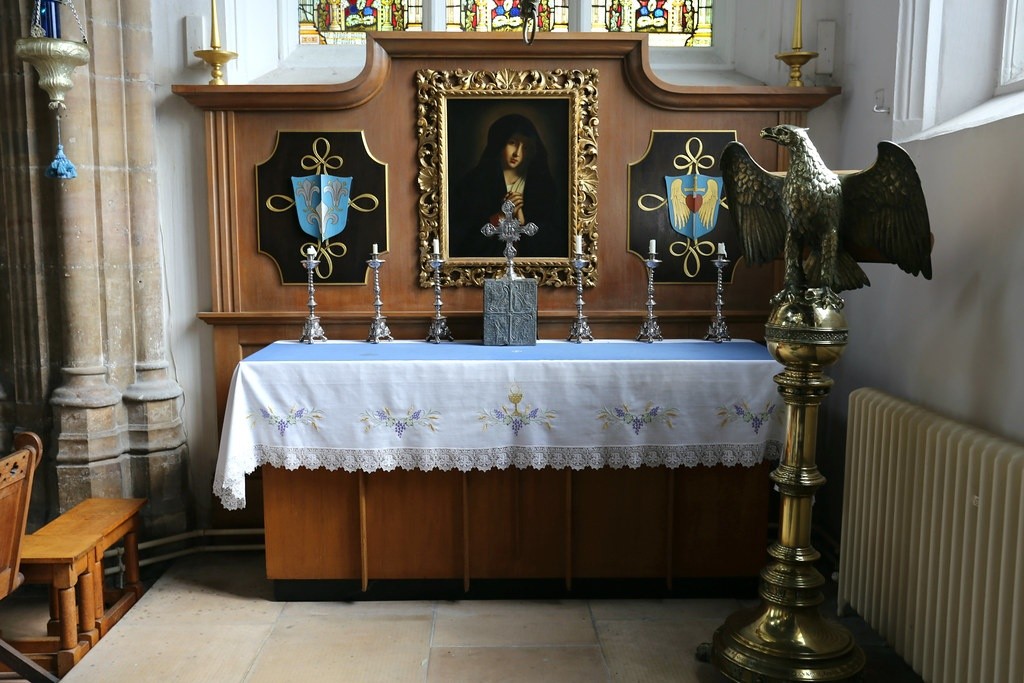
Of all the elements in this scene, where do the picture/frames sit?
[414,68,600,289]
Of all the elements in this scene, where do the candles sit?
[718,242,727,258]
[307,247,316,254]
[576,232,582,253]
[433,237,439,254]
[373,243,378,254]
[649,239,656,253]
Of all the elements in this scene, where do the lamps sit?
[15,0,91,180]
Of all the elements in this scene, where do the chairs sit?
[0,431,60,683]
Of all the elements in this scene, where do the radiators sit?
[836,387,1024,683]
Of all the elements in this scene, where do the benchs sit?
[0,498,150,678]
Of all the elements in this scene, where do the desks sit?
[212,339,787,593]
